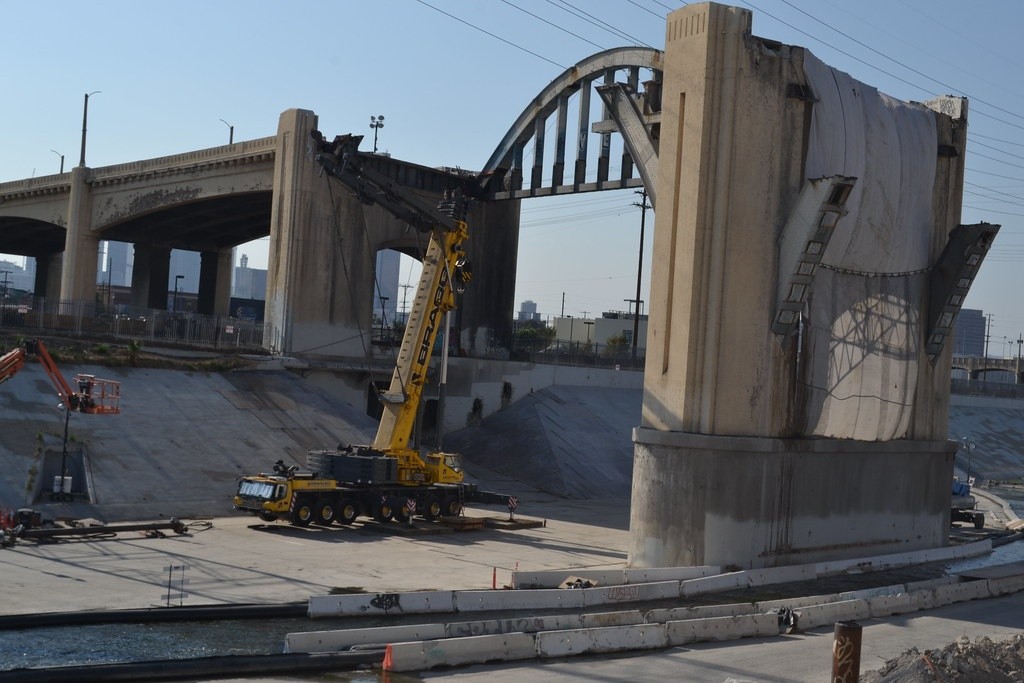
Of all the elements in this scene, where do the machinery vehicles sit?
[0,338,123,502]
[235,130,473,530]
[954,435,988,529]
